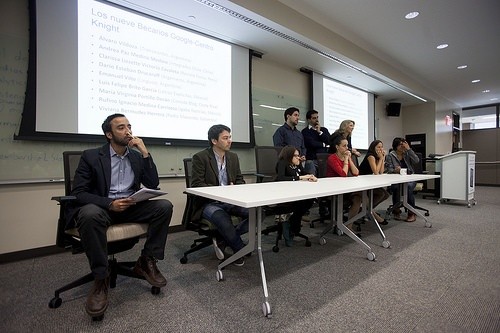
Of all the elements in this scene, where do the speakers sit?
[387,103,401,116]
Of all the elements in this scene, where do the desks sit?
[186,172,441,319]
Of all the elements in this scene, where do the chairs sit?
[179,146,430,264]
[47,150,160,321]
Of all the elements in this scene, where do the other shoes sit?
[283,222,294,238]
[394,213,402,221]
[233,252,244,266]
[242,246,252,257]
[356,233,361,237]
[407,213,416,223]
[380,220,388,225]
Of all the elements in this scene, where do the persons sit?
[272,107,306,161]
[384,137,421,222]
[326,136,389,237]
[359,140,389,222]
[71,113,173,316]
[302,110,331,159]
[329,120,360,157]
[182,124,265,267]
[274,145,317,241]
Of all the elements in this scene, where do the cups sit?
[400,168,407,175]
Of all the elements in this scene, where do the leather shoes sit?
[86,277,110,317]
[135,256,167,288]
